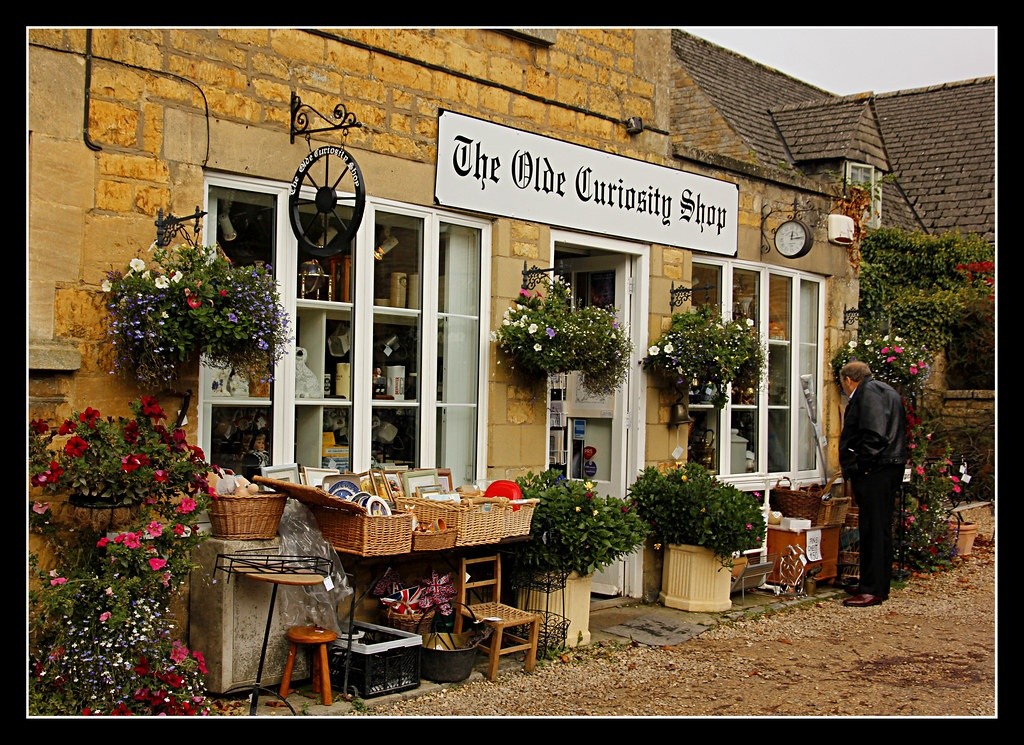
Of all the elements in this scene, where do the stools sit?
[277,625,336,706]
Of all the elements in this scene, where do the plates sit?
[327,481,392,516]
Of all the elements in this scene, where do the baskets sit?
[773,476,822,527]
[325,620,424,700]
[846,506,860,530]
[842,551,860,579]
[819,471,847,525]
[385,607,435,634]
[203,475,541,557]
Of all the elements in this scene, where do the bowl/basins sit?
[375,299,390,306]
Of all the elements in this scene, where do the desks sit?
[233,562,323,717]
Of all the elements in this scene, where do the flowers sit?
[26,242,297,716]
[485,259,997,580]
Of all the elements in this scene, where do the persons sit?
[243,433,269,482]
[839,361,907,606]
[374,366,386,395]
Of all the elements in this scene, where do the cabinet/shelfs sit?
[767,523,842,593]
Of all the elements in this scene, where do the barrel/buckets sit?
[415,602,479,683]
[484,480,522,511]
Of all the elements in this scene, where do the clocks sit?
[760,198,823,262]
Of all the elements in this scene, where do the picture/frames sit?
[261,463,301,492]
[300,466,453,504]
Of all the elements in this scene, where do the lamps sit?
[374,236,399,260]
[218,208,237,241]
[627,117,643,136]
[296,259,329,277]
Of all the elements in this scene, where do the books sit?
[550,429,564,464]
[550,399,567,427]
[548,369,565,389]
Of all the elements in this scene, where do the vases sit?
[946,520,976,557]
[210,356,234,398]
[69,493,140,533]
[659,543,732,612]
[516,570,591,649]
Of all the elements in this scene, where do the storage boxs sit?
[327,619,423,700]
[321,432,349,473]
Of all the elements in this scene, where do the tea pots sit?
[694,428,716,470]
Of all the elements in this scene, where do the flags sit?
[379,586,422,615]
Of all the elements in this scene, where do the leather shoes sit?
[844,594,883,606]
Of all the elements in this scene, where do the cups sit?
[390,272,407,307]
[427,518,447,532]
[408,275,419,309]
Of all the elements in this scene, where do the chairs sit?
[730,552,778,604]
[452,553,540,683]
